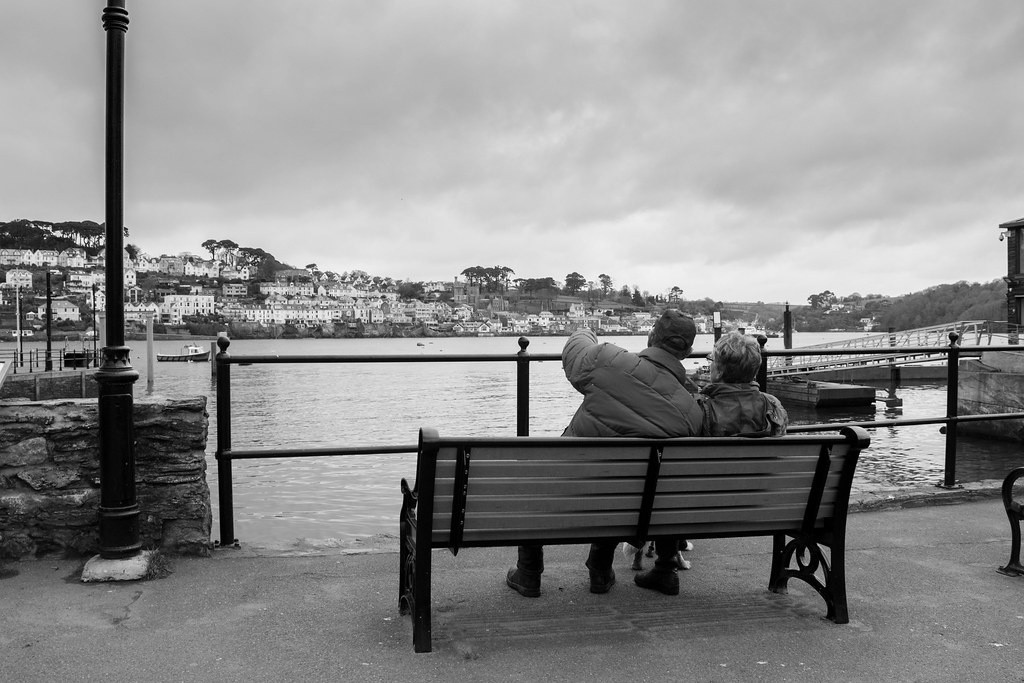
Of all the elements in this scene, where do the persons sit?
[506,306,703,597]
[631,330,789,596]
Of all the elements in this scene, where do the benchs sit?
[399,425,872,654]
[999,461,1024,578]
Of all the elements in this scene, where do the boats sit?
[157,345,210,362]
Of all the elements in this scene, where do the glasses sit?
[706,354,715,363]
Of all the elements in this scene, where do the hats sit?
[653,309,696,352]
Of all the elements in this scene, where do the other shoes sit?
[591,568,616,593]
[508,567,542,598]
[634,568,680,596]
